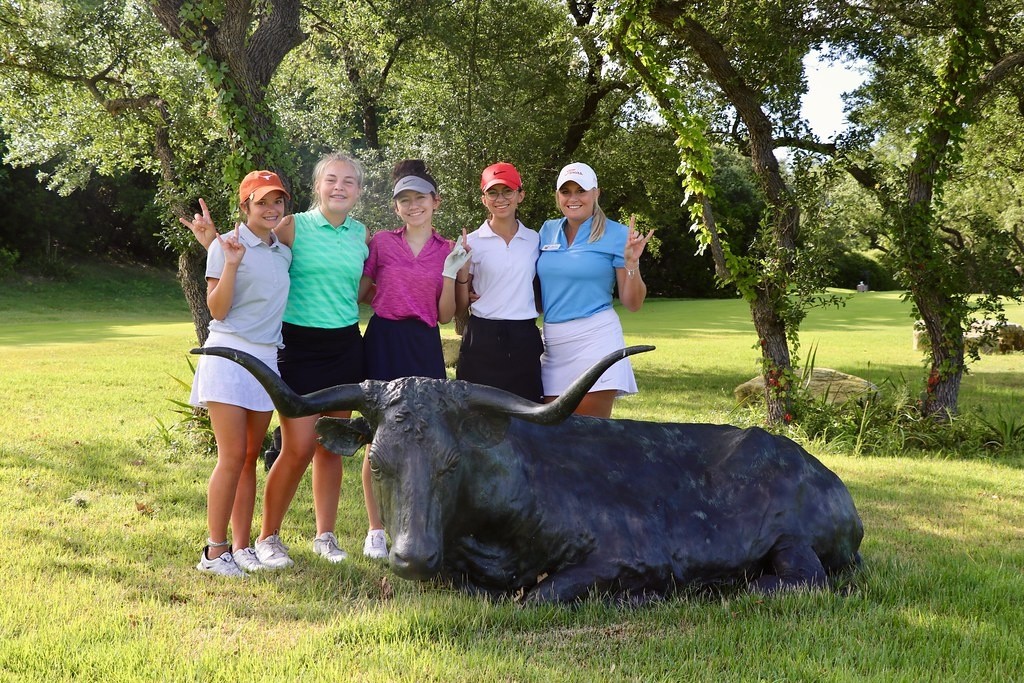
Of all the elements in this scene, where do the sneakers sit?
[195,544,250,577]
[254,529,295,569]
[228,543,267,571]
[312,531,347,564]
[363,529,389,558]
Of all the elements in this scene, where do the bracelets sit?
[624,264,641,280]
[458,281,468,285]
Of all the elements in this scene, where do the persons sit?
[456,162,545,406]
[178,153,377,567]
[358,159,474,558]
[469,160,654,418]
[187,170,293,577]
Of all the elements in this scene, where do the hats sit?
[556,161,597,191]
[392,175,437,199]
[480,162,521,193]
[239,170,290,204]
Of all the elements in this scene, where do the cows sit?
[188,342,870,610]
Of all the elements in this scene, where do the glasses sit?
[484,187,520,201]
